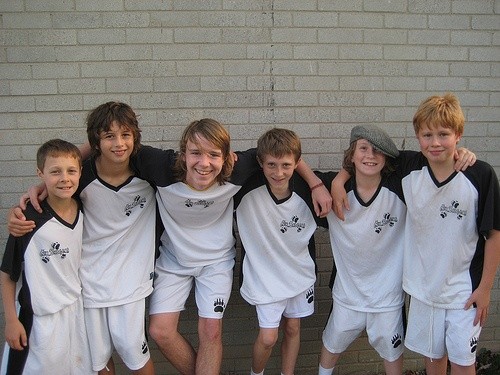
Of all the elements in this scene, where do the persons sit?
[76,118,332,375]
[318,121,475,375]
[1,139,96,375]
[330,93,499,375]
[231,127,320,375]
[22,103,239,375]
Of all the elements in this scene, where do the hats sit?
[350,125,400,159]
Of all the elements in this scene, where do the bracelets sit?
[312,182,324,191]
